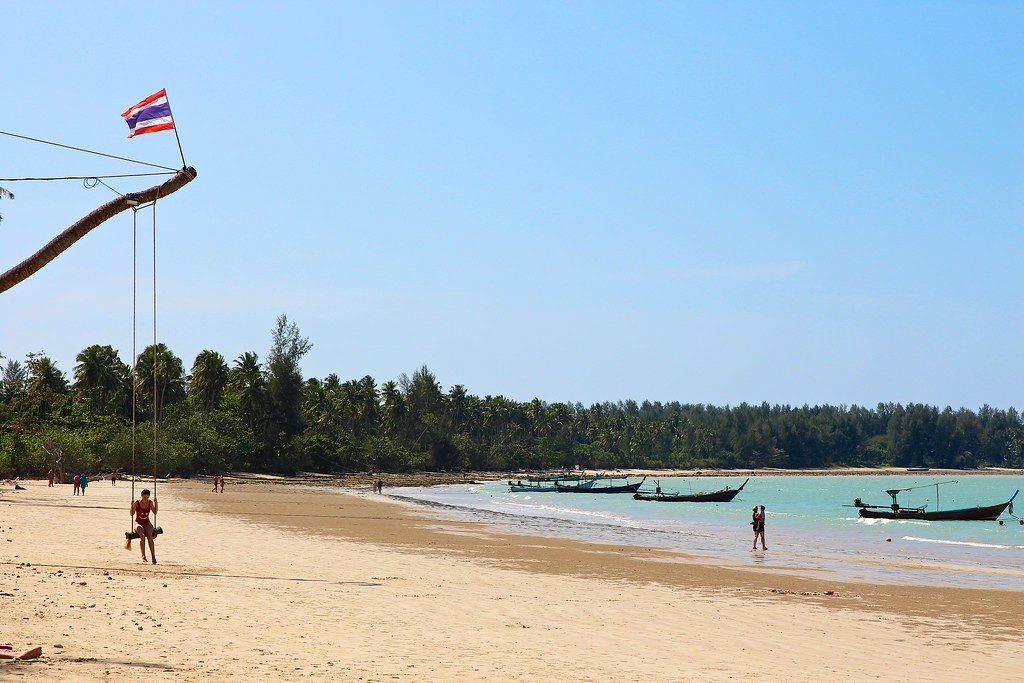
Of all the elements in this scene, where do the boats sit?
[633,477,750,502]
[508,471,646,494]
[842,480,1020,521]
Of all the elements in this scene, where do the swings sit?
[124,199,164,540]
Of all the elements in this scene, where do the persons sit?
[749,504,769,551]
[111,470,117,486]
[218,474,225,493]
[130,488,158,564]
[211,474,218,492]
[371,478,384,495]
[48,469,55,487]
[73,474,88,496]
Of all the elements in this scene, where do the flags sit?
[119,87,177,140]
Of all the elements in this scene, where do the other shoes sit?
[752,547,757,549]
[763,547,767,550]
[152,559,157,564]
[142,556,148,562]
[756,526,759,530]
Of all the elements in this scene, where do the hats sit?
[753,506,758,511]
